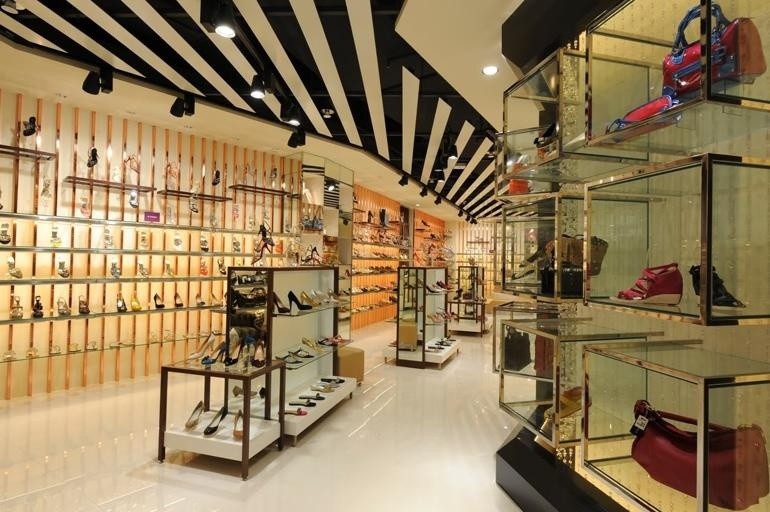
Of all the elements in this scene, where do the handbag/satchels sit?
[505,326,531,371]
[662,2,766,104]
[540,237,608,298]
[616,95,681,142]
[631,400,769,510]
[299,203,324,230]
[509,154,529,195]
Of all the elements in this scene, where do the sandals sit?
[189,194,198,212]
[10,295,90,321]
[81,194,91,217]
[428,311,454,323]
[0,221,241,279]
[275,335,345,363]
[543,386,592,419]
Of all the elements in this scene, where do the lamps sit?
[252,68,275,99]
[23,117,36,136]
[83,67,113,96]
[1,1,20,14]
[288,127,305,148]
[201,2,235,38]
[281,102,302,127]
[398,143,481,224]
[321,105,334,118]
[171,94,194,117]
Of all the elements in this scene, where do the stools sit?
[337,346,364,386]
[398,321,417,350]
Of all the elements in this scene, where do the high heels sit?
[196,292,222,306]
[427,281,453,293]
[258,221,274,246]
[609,263,683,305]
[124,154,139,172]
[273,289,349,315]
[165,161,177,177]
[87,147,100,167]
[187,333,265,367]
[689,264,746,307]
[305,245,329,266]
[23,117,39,136]
[251,239,271,265]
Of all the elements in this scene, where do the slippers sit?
[129,190,139,207]
[425,338,456,352]
[278,378,345,415]
[212,169,220,185]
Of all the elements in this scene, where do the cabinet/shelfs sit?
[156,354,287,481]
[270,266,357,448]
[491,1,770,510]
[281,152,355,345]
[425,266,462,369]
[232,280,267,340]
[1,142,293,364]
[351,200,412,315]
[415,229,451,267]
[448,265,494,338]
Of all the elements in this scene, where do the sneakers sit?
[351,226,408,315]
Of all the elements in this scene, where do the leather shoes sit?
[204,407,227,434]
[174,293,183,307]
[260,387,265,398]
[233,385,257,396]
[534,123,556,148]
[234,410,243,438]
[186,401,204,428]
[154,293,164,308]
[117,291,142,311]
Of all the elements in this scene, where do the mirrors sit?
[398,268,424,363]
[226,267,269,363]
